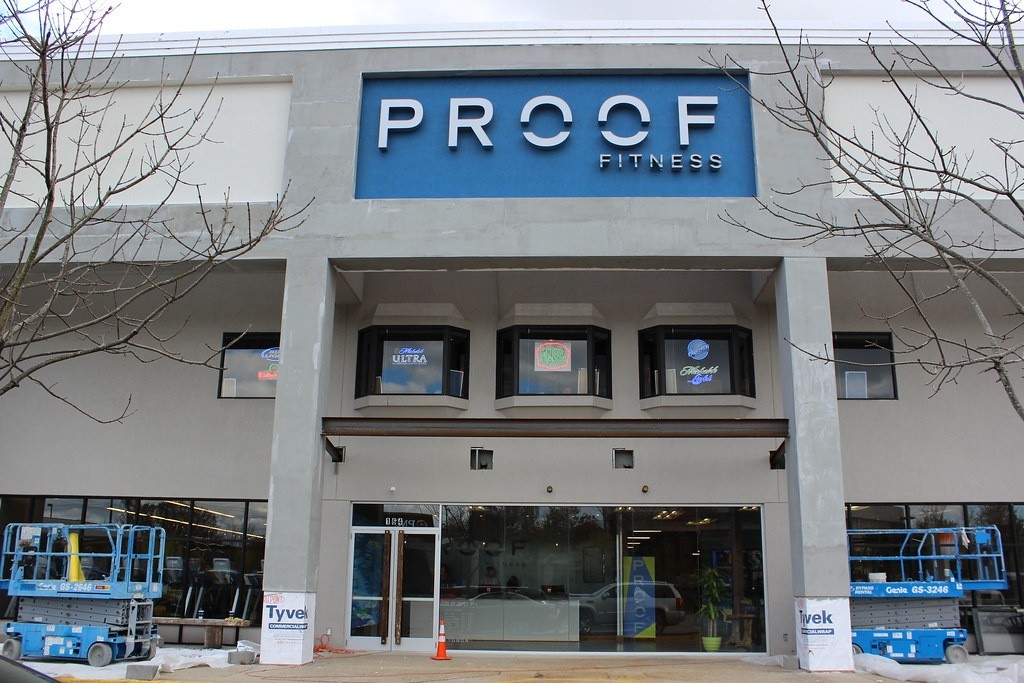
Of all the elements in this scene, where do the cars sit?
[452,591,551,607]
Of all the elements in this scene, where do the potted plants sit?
[698,567,729,652]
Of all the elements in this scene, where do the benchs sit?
[152,617,251,650]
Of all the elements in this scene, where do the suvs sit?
[566,580,686,638]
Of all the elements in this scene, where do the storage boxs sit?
[869,573,887,583]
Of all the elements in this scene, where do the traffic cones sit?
[431,620,453,660]
